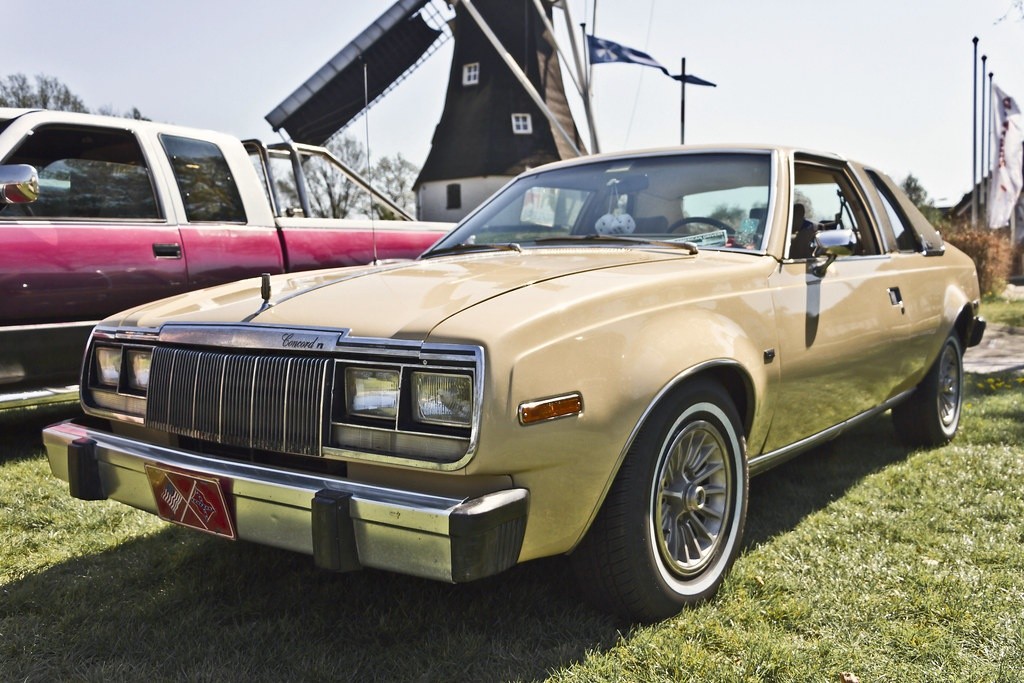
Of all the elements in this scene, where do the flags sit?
[984,84,1024,229]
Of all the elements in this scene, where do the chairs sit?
[753,202,814,258]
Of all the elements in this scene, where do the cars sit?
[39,138,987,623]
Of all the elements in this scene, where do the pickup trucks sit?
[0,111,478,431]
